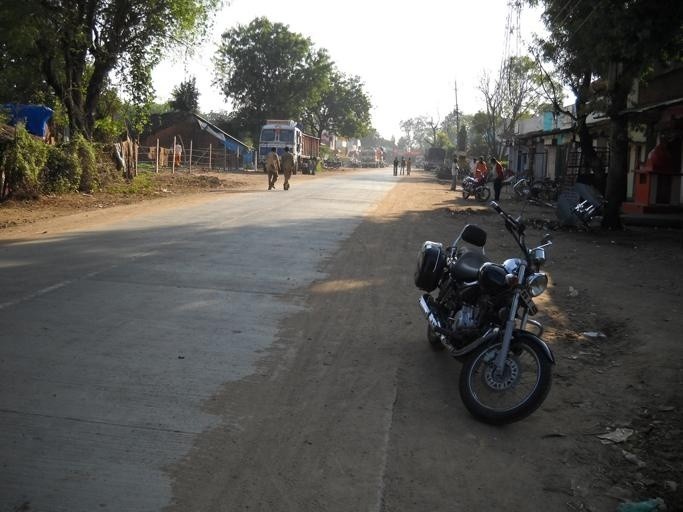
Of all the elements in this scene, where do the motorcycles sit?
[462,174,491,202]
[414,200,556,425]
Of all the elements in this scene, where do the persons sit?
[266,146,281,191]
[393,156,398,176]
[449,158,459,191]
[406,157,412,176]
[469,156,487,200]
[491,157,506,202]
[281,146,295,190]
[174,140,182,168]
[399,156,405,176]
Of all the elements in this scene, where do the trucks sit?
[424,148,444,170]
[258,120,320,174]
[361,149,383,168]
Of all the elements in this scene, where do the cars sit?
[416,156,425,168]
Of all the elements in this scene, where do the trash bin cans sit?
[631,169,657,206]
[302,158,315,175]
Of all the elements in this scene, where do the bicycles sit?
[529,176,563,201]
